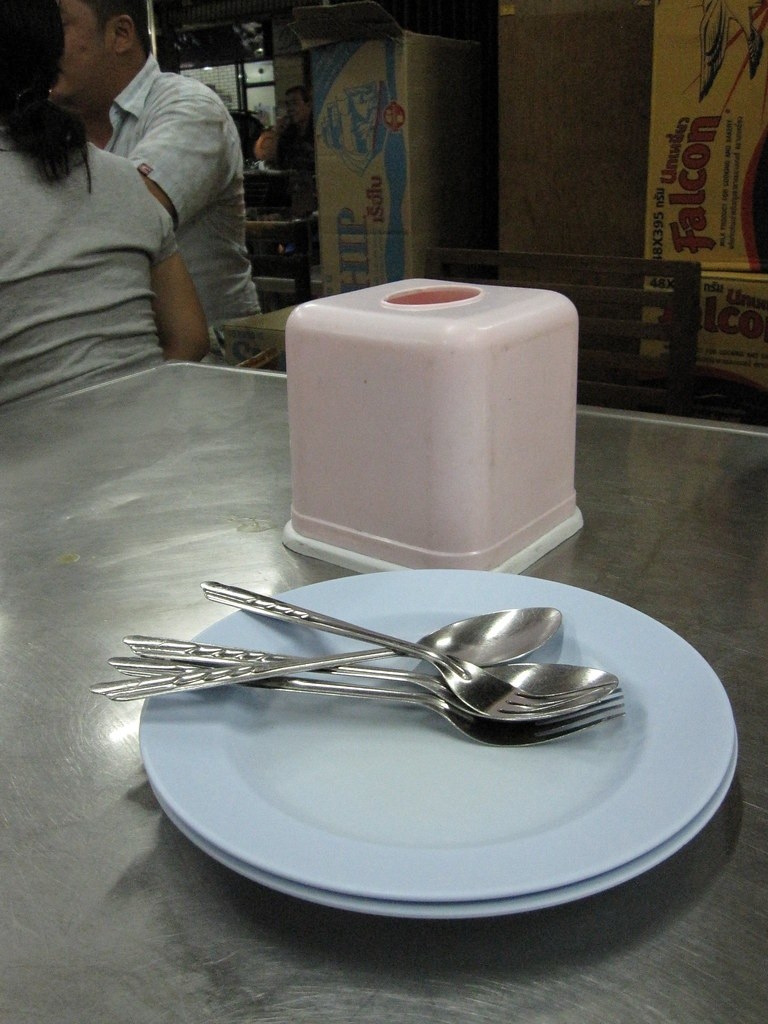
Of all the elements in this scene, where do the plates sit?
[140,568,737,918]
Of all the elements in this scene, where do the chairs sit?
[418,244,703,418]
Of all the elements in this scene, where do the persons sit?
[0,0,212,413]
[48,1,264,368]
[242,85,316,263]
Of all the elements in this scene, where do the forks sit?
[117,668,625,746]
[200,579,615,721]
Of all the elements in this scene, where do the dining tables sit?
[0,360,767,1023]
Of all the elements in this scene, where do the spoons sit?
[124,635,619,719]
[92,607,561,701]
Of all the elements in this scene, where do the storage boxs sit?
[217,303,303,374]
[287,1,498,299]
